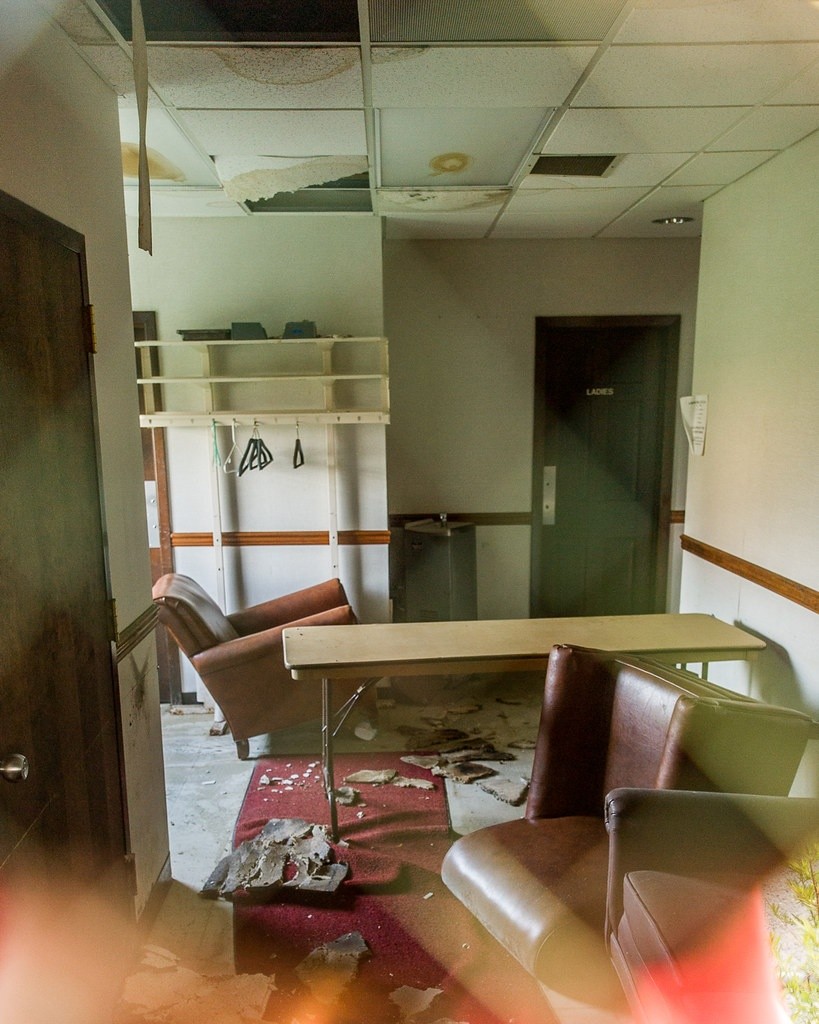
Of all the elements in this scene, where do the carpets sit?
[223,751,510,1024]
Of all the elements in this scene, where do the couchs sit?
[440,644,819,1007]
[604,787,819,1024]
[152,573,379,761]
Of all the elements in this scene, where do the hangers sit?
[292,424,304,469]
[211,418,273,477]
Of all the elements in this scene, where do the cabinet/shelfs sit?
[135,337,390,724]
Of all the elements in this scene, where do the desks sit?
[282,613,767,844]
